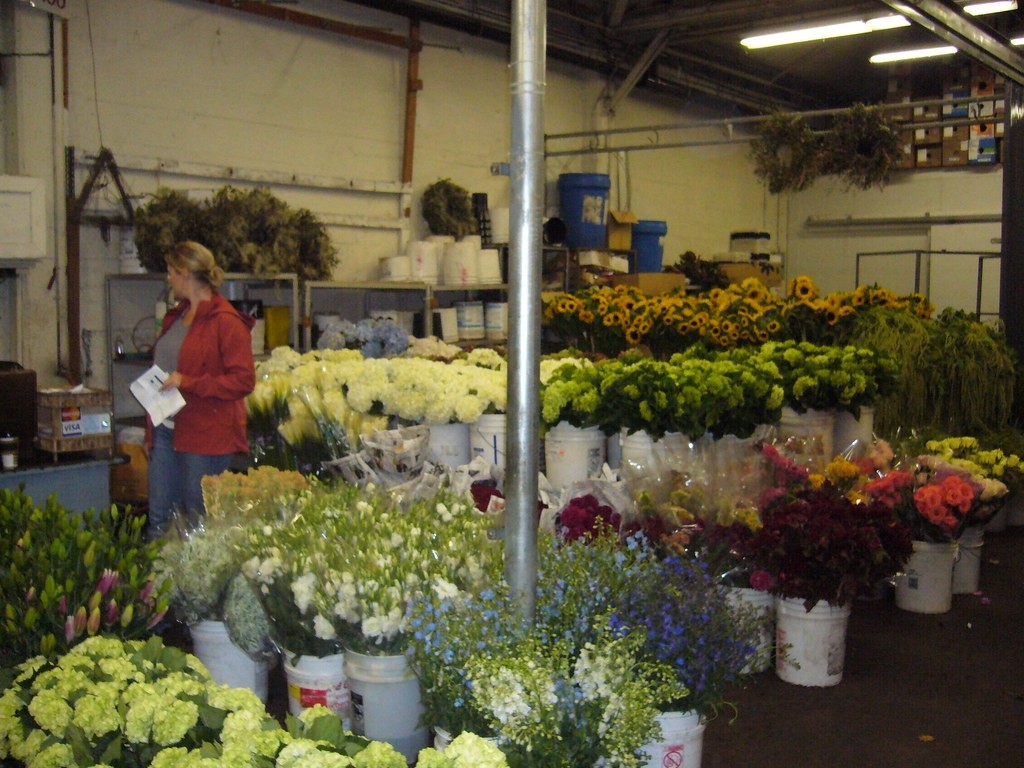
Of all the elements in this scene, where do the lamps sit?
[740,0,1019,49]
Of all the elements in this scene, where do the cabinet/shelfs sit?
[105,243,569,524]
[37,385,113,463]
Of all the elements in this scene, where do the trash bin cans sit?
[629,218,667,273]
[557,171,610,249]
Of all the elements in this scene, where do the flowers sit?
[133,187,339,280]
[0,274,1023,767]
[746,101,904,196]
[420,179,479,242]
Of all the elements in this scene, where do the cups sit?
[0,437,19,470]
[379,235,502,284]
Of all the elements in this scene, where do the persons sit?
[129,239,256,551]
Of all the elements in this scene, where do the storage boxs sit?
[578,250,628,273]
[715,231,782,290]
[608,209,638,251]
[613,273,684,296]
[887,56,1004,170]
